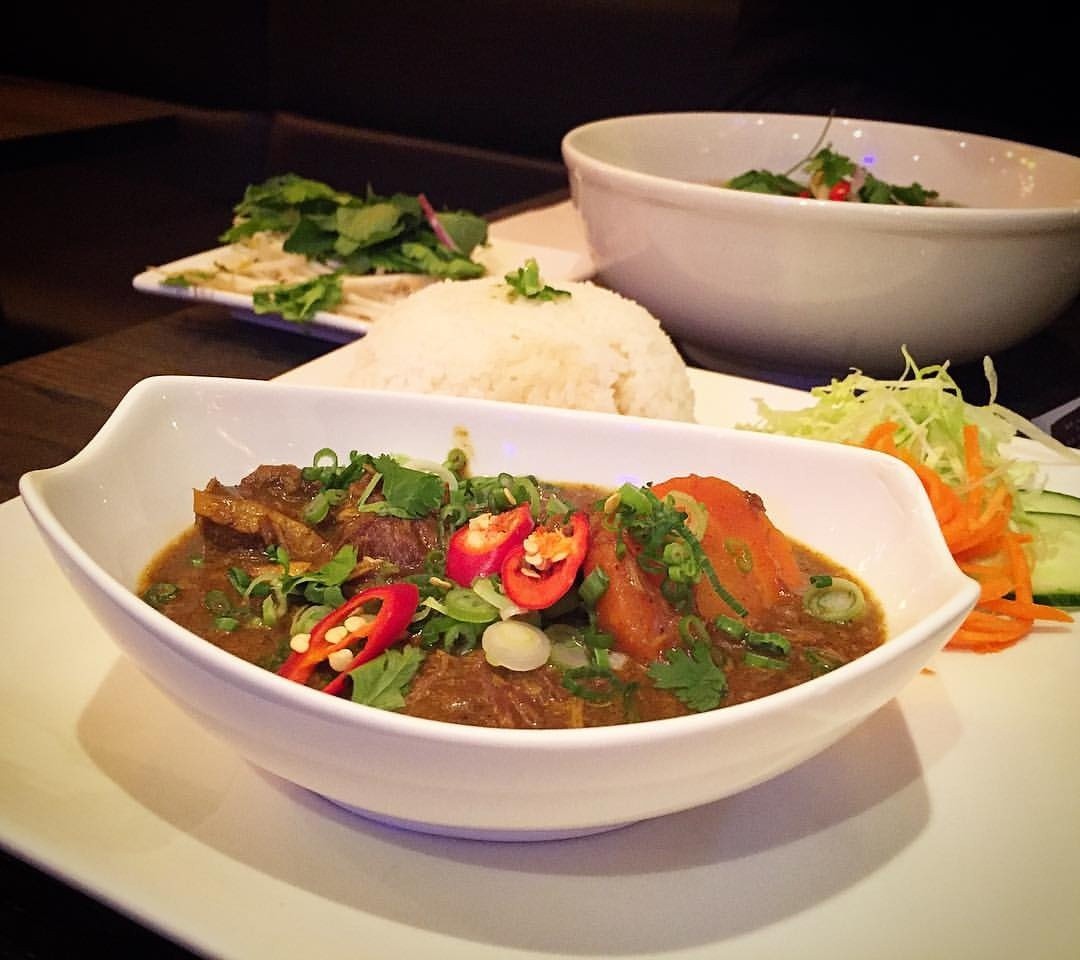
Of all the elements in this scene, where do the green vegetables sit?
[211,168,491,323]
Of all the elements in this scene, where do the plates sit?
[0,307,1080,958]
[132,206,595,352]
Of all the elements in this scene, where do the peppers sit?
[493,514,596,609]
[270,577,421,700]
[442,507,535,603]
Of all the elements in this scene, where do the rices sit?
[357,263,700,427]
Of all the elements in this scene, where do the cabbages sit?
[719,338,1080,536]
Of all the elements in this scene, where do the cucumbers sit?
[968,478,1080,608]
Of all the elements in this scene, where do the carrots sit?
[837,414,1076,669]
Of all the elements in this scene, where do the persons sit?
[718,0,1079,151]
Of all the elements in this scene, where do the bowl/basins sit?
[14,368,986,854]
[559,110,1080,420]
